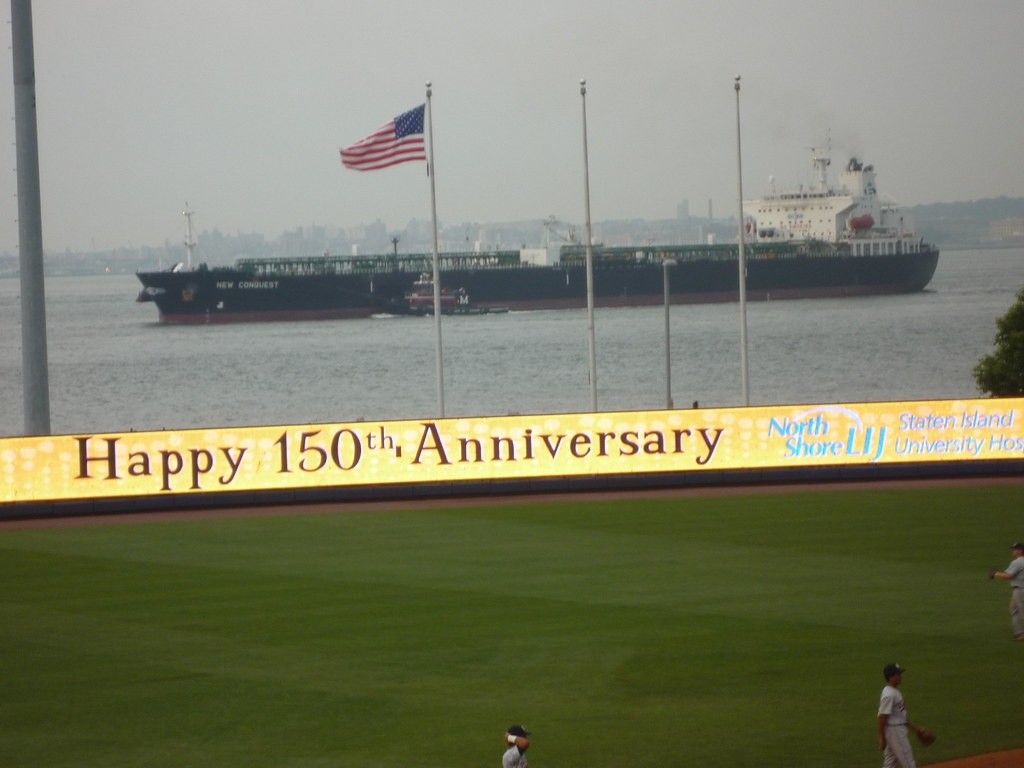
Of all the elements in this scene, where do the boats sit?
[134,128,940,324]
[368,272,509,315]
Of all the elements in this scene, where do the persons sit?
[502,724,532,768]
[876,663,937,768]
[989,542,1024,642]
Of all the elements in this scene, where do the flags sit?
[338,103,426,171]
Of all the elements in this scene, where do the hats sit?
[884,663,905,675]
[508,724,531,737]
[1011,541,1024,549]
